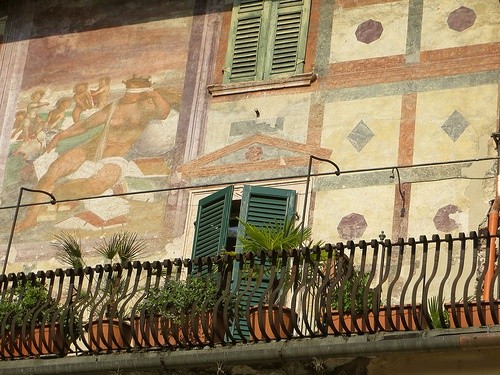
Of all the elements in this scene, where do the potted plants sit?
[127,272,246,350]
[0,278,88,355]
[325,269,427,333]
[212,213,325,343]
[49,230,153,351]
